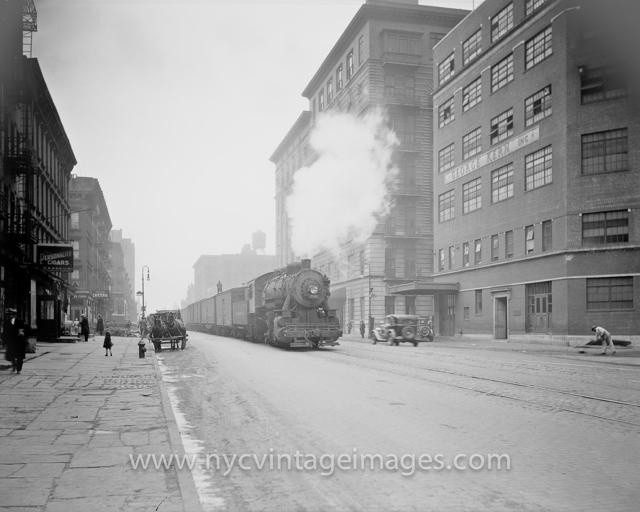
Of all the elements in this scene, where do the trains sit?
[181,259,343,351]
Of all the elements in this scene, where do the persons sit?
[102,331,115,356]
[591,324,617,355]
[95,312,104,335]
[1,306,28,375]
[346,321,352,334]
[78,313,91,341]
[359,320,366,338]
[216,280,223,294]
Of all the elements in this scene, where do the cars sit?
[370,315,433,346]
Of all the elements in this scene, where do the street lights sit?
[136,265,150,337]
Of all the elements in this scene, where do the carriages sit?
[147,309,188,351]
